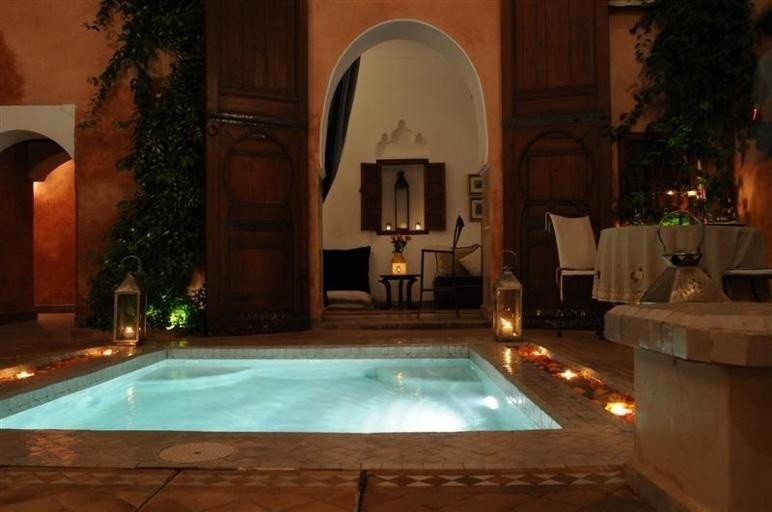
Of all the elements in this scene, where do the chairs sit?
[544,209,617,341]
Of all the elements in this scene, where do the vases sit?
[392,251,406,262]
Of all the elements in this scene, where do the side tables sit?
[379,273,420,310]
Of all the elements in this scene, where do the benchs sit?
[433,277,483,310]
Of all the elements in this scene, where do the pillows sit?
[326,291,373,310]
[322,245,371,304]
[435,244,481,278]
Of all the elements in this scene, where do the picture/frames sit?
[469,199,483,222]
[469,175,483,196]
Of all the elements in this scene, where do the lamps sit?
[415,222,421,231]
[492,251,525,343]
[113,254,145,347]
[391,262,407,275]
[386,222,391,231]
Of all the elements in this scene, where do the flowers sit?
[390,234,412,250]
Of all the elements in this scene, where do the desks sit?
[593,224,756,307]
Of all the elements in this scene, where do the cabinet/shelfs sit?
[359,159,445,236]
